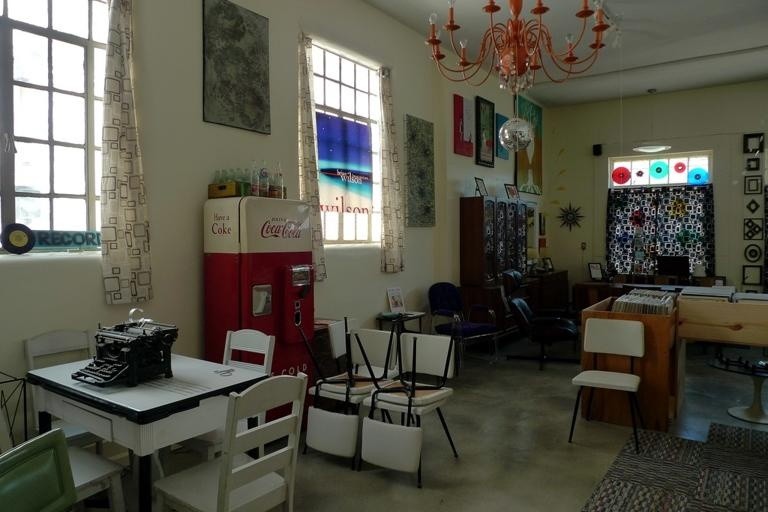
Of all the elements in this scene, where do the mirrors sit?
[526,206,536,251]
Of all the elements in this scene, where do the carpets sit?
[580,422,768,512]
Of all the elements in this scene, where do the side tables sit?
[377,313,427,332]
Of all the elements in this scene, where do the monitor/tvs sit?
[657,256,689,276]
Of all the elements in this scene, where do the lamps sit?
[424,0,614,154]
[630,89,672,156]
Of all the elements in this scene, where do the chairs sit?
[698,340,768,426]
[152,371,310,512]
[298,316,397,462]
[428,281,500,370]
[362,332,457,489]
[306,326,398,473]
[355,320,457,474]
[0,427,77,512]
[0,413,126,510]
[568,316,648,456]
[157,327,276,467]
[506,294,577,373]
[23,328,105,462]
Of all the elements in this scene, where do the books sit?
[611,288,678,316]
[680,285,768,304]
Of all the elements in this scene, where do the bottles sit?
[251,160,259,196]
[212,167,242,182]
[270,159,283,199]
[241,168,251,196]
[259,160,269,197]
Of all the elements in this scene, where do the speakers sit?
[593,144,602,156]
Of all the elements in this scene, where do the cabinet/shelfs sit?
[675,287,768,420]
[458,196,543,321]
[581,295,677,431]
[530,269,571,311]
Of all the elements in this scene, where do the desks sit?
[574,280,710,328]
[24,353,272,512]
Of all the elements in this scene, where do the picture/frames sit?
[495,112,512,160]
[512,89,544,196]
[539,212,546,236]
[542,257,554,272]
[474,95,496,168]
[473,177,490,194]
[403,113,438,229]
[452,91,477,159]
[202,0,273,135]
[588,262,602,279]
[503,183,520,202]
